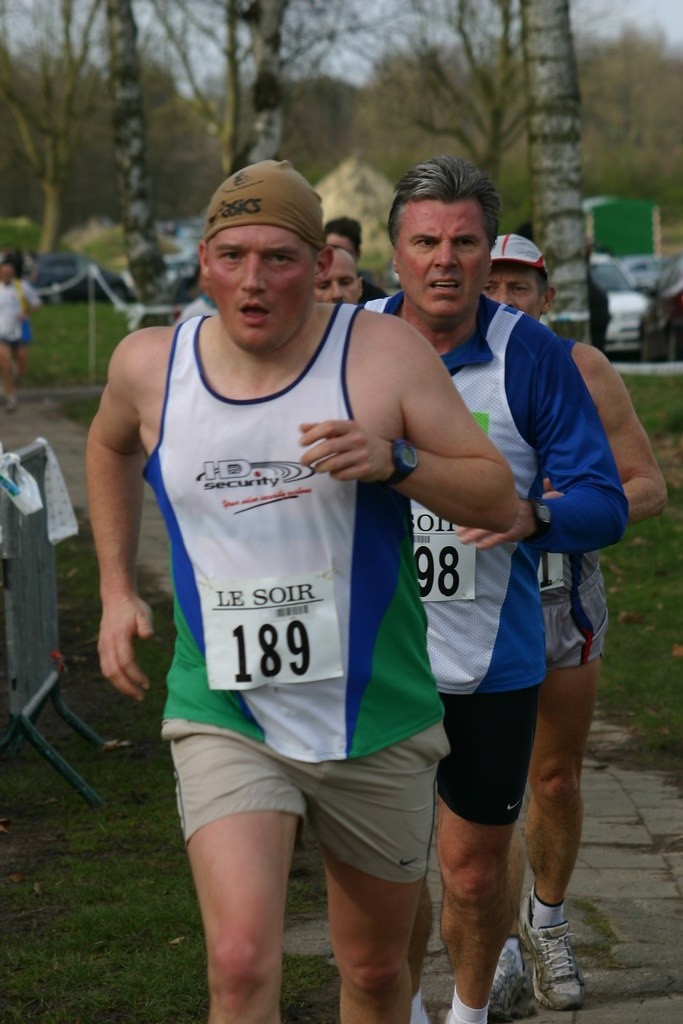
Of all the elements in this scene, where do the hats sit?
[204,159,323,247]
[490,234,548,278]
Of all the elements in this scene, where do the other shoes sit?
[7,395,17,411]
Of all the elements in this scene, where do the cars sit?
[631,254,682,361]
[27,253,128,305]
[585,249,671,354]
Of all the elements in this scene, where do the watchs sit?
[383,438,418,486]
[520,498,550,544]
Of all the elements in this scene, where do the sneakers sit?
[518,895,583,1010]
[487,947,523,1019]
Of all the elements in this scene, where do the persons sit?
[482,232,667,1018]
[174,266,219,326]
[312,216,389,305]
[83,159,519,1024]
[0,249,40,412]
[357,152,630,1024]
